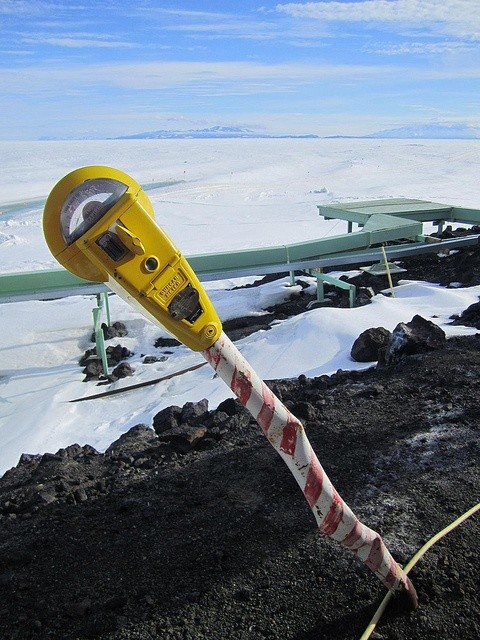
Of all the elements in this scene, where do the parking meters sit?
[43,165,419,610]
